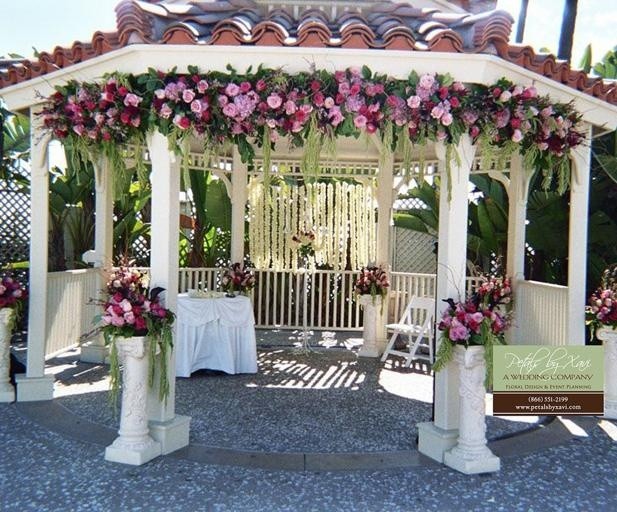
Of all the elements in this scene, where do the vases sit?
[357,294,381,357]
[131,326,148,337]
[224,279,236,298]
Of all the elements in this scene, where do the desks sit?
[174,291,257,378]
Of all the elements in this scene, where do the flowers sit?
[356,268,388,298]
[468,332,489,345]
[0,273,28,312]
[440,276,515,345]
[585,266,617,330]
[221,263,257,292]
[97,268,173,410]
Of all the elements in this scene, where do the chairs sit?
[381,296,436,367]
